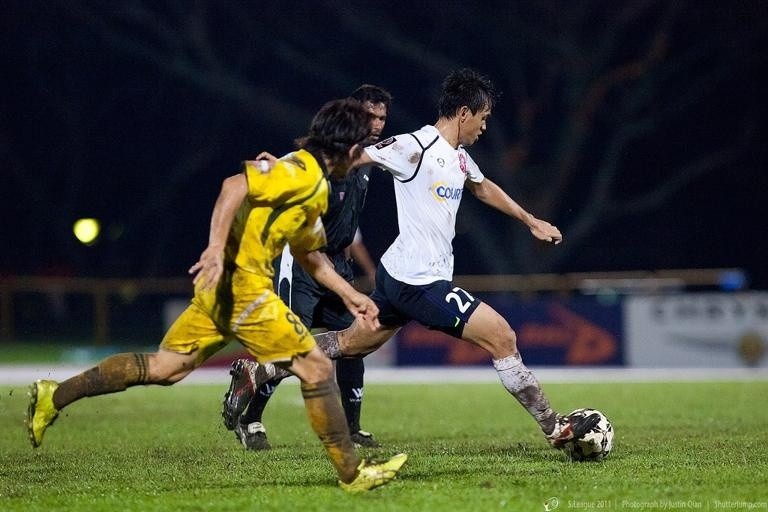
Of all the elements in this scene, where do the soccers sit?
[560,409,614,462]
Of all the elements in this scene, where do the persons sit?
[219,66,601,452]
[25,97,410,497]
[231,84,395,454]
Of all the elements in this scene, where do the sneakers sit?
[233,414,271,450]
[222,358,259,430]
[349,429,378,449]
[339,453,408,493]
[25,378,59,448]
[543,414,600,450]
